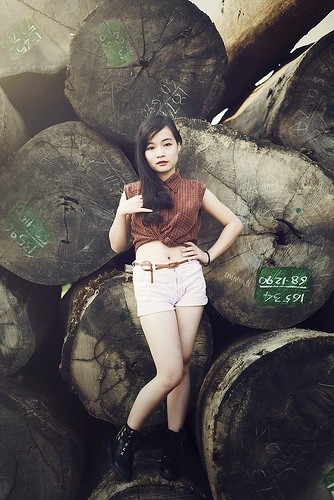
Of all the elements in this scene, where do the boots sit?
[107,424,141,482]
[159,427,188,482]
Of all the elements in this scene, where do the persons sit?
[106,114,245,482]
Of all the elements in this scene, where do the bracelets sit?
[202,251,210,268]
